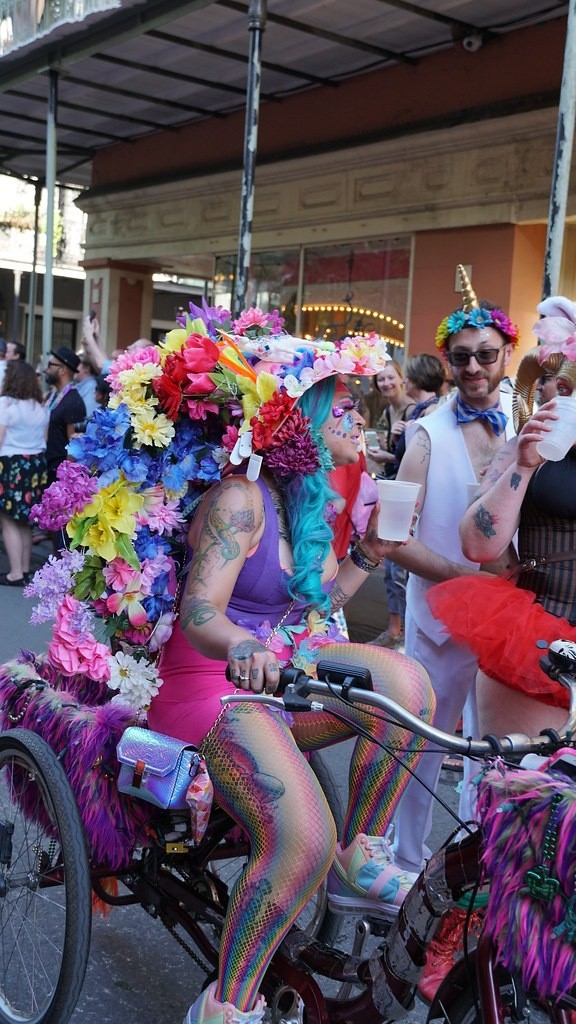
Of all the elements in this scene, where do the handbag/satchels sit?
[115,726,205,809]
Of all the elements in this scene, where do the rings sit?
[238,675,249,683]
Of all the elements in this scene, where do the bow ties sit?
[456,393,509,437]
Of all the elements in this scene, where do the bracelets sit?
[349,543,381,573]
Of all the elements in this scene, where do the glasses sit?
[445,343,506,364]
[48,361,62,368]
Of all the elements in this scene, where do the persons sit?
[356,355,417,482]
[381,308,525,872]
[145,338,438,1023]
[365,354,447,653]
[0,316,153,588]
[455,346,576,740]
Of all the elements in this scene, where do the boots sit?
[417,907,486,1007]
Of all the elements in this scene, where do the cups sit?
[376,479,422,542]
[536,397,576,463]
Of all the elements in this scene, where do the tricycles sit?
[0,661,575,1024]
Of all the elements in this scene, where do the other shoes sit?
[24,571,33,582]
[0,575,26,586]
[366,631,404,650]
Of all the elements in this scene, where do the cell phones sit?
[90,311,96,321]
[364,431,380,450]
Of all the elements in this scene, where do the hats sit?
[50,346,81,373]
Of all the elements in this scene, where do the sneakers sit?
[327,823,420,922]
[182,979,267,1024]
